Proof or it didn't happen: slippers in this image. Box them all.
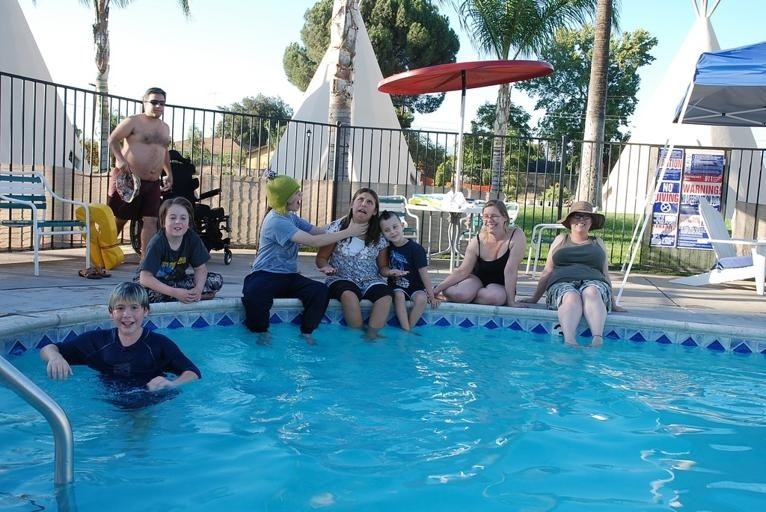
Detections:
[78,264,112,280]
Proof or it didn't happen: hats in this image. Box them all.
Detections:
[115,168,142,204]
[560,200,605,232]
[263,167,300,219]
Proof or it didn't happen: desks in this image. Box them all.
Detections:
[405,202,479,273]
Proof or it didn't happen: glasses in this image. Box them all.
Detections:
[146,98,166,107]
[570,212,592,220]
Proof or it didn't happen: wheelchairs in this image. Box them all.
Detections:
[129,150,232,265]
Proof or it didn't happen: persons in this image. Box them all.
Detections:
[378,209,442,336]
[104,86,175,263]
[242,169,370,345]
[315,186,413,343]
[433,199,531,309]
[39,280,202,412]
[129,198,225,304]
[515,200,632,346]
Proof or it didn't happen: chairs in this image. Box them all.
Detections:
[0,170,91,276]
[377,194,422,245]
[456,201,520,268]
[696,198,766,295]
[526,204,597,279]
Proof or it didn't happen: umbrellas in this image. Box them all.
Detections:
[375,59,555,272]
